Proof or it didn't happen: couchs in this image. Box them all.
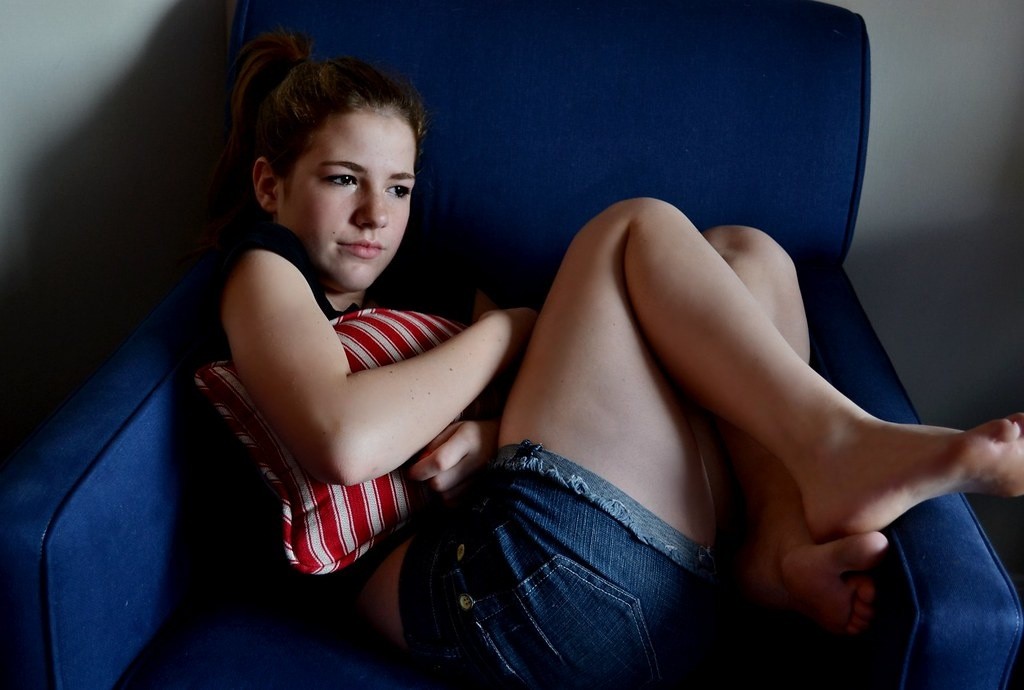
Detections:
[0,0,1024,690]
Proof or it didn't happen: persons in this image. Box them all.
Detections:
[181,32,1024,690]
